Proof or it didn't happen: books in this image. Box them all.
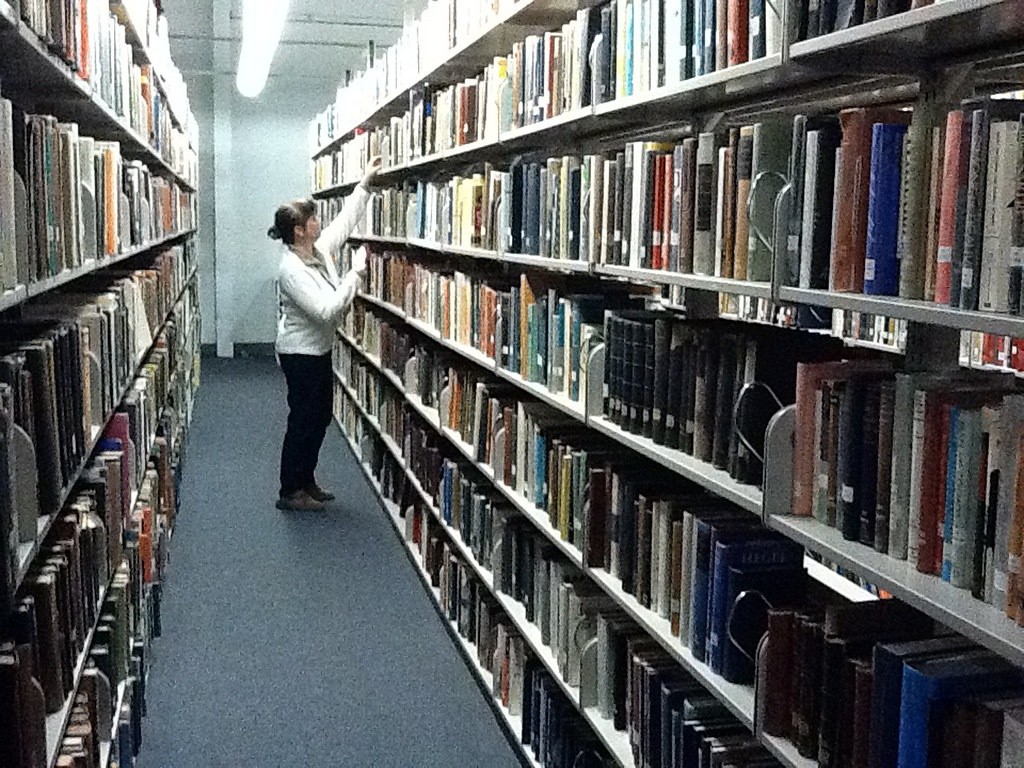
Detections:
[312,110,409,193]
[319,107,1024,318]
[311,0,527,157]
[408,0,944,158]
[0,0,203,768]
[333,241,1024,768]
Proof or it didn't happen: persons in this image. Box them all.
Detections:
[266,156,382,512]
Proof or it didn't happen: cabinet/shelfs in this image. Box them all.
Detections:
[0,0,200,768]
[309,0,1024,768]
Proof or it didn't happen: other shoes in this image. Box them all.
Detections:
[307,483,334,501]
[276,488,324,511]
